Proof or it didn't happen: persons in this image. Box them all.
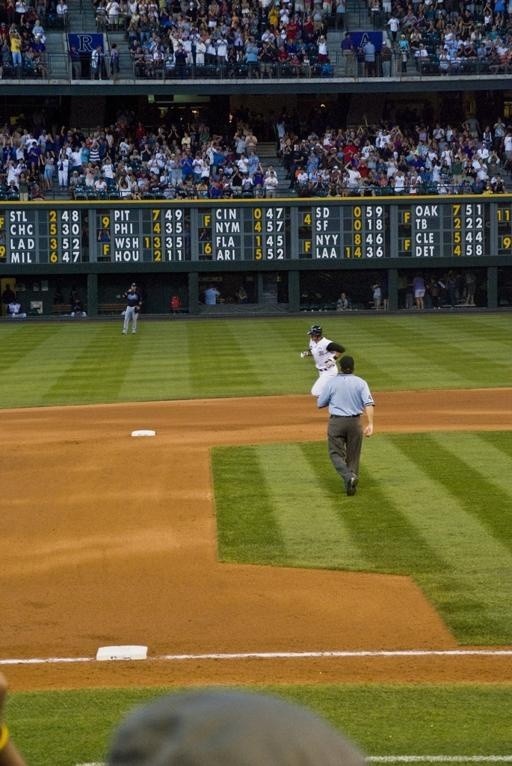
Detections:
[0,671,26,766]
[300,325,345,398]
[1,1,512,80]
[2,266,511,335]
[1,95,512,202]
[108,690,366,766]
[318,356,376,496]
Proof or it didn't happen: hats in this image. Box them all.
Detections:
[131,282,136,286]
[339,356,354,368]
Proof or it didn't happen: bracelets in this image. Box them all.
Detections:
[0,720,10,753]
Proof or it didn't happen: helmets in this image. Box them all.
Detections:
[306,325,322,336]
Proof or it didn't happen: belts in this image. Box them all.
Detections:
[319,368,327,371]
[330,414,359,419]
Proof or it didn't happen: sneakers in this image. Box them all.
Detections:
[121,330,137,334]
[347,472,359,496]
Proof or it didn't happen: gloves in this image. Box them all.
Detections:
[325,358,336,367]
[301,351,309,359]
